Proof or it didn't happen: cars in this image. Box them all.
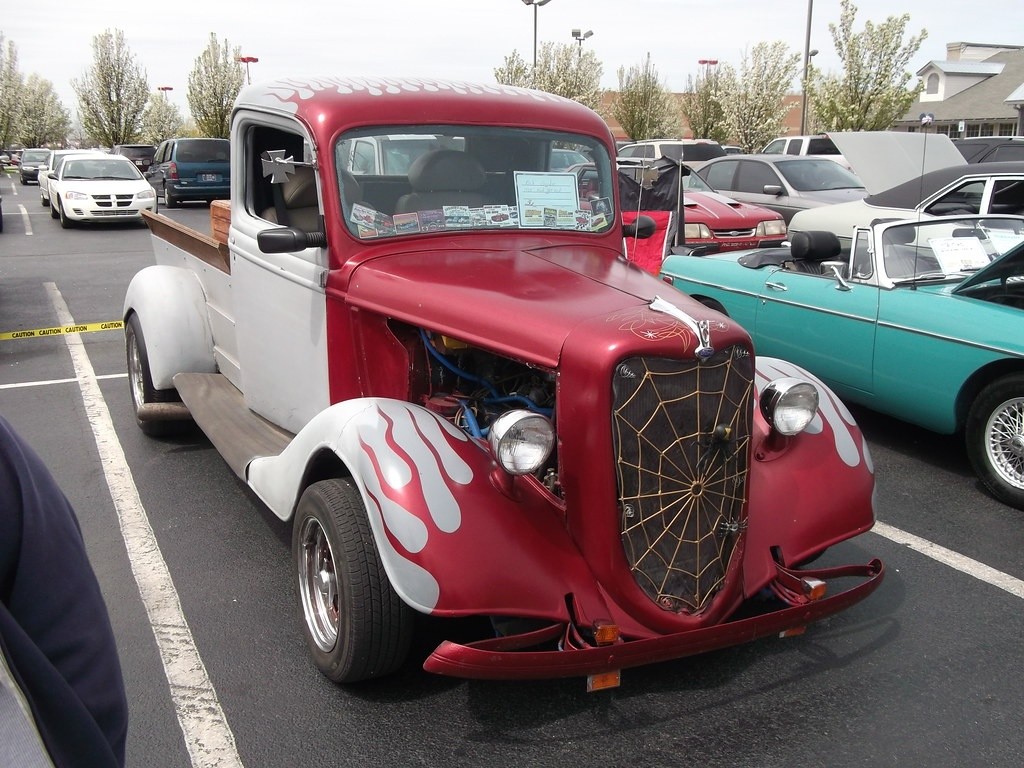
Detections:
[550,128,1024,234]
[45,152,159,228]
[785,161,1024,260]
[561,156,790,262]
[9,141,157,207]
[338,133,466,176]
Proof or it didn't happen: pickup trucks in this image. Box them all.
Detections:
[108,76,886,692]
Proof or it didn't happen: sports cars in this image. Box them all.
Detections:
[654,211,1024,511]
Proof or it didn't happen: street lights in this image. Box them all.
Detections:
[237,56,259,86]
[521,0,553,84]
[570,28,595,94]
[804,48,820,135]
[157,86,174,104]
[698,58,719,82]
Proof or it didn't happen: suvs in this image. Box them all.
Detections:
[142,137,230,210]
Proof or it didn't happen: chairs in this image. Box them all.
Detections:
[263,166,376,233]
[70,163,84,175]
[873,217,932,278]
[786,231,843,276]
[395,149,496,215]
